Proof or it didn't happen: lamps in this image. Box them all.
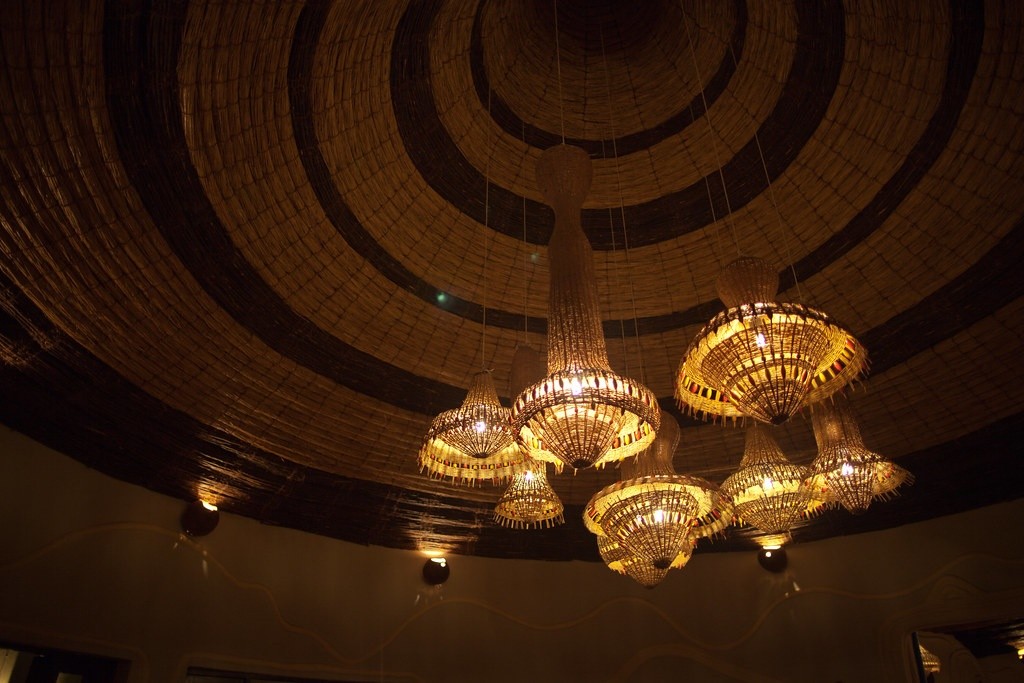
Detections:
[582,17,740,589]
[758,546,787,574]
[686,94,830,534]
[492,108,565,530]
[721,21,915,515]
[183,500,220,537]
[422,559,449,586]
[417,54,526,490]
[674,0,871,430]
[508,0,663,474]
